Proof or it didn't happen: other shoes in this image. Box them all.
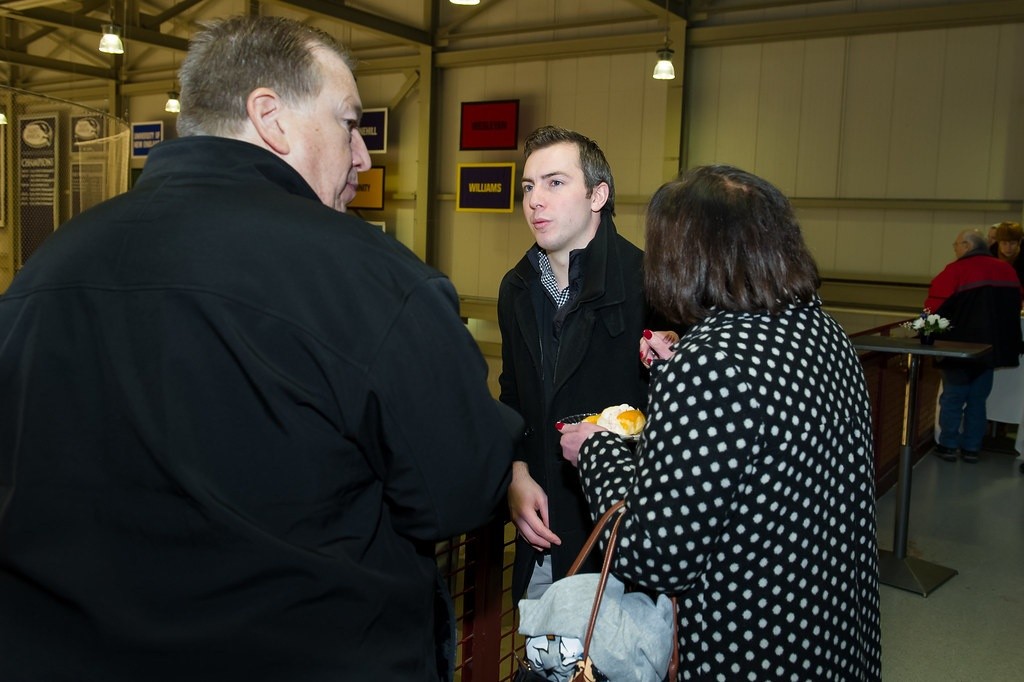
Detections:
[959,452,979,464]
[932,444,957,462]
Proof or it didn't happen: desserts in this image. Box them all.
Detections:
[582,404,647,437]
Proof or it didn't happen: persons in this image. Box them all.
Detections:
[554,166,881,681]
[0,17,518,682]
[924,221,1024,463]
[500,129,683,612]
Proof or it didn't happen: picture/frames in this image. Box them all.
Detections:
[455,162,515,213]
[345,165,385,210]
[458,99,518,152]
[356,107,388,154]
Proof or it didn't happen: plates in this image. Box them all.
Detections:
[558,412,601,426]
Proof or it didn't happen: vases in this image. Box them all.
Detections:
[920,331,937,345]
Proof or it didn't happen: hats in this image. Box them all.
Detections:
[987,222,1024,241]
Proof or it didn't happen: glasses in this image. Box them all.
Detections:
[952,241,967,248]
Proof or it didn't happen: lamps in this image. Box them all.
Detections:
[165,0,181,112]
[652,1,675,79]
[0,113,8,125]
[99,0,124,54]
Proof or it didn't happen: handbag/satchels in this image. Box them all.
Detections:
[511,500,679,682]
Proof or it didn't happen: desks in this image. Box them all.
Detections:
[844,343,992,597]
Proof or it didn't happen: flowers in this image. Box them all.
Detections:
[900,309,956,336]
